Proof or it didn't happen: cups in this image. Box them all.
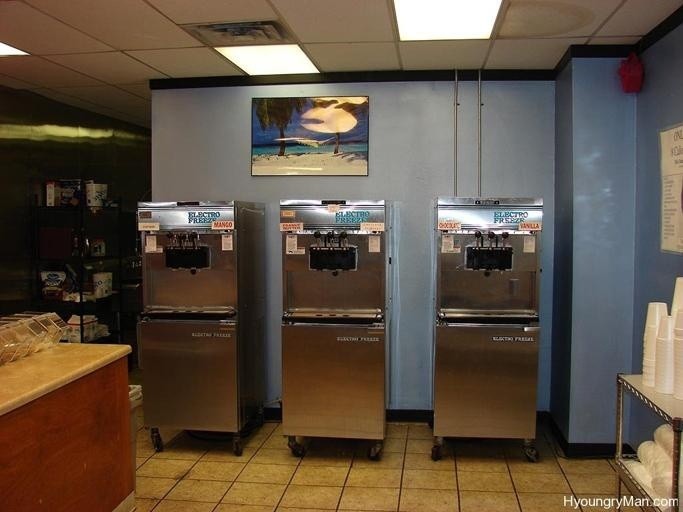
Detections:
[643,277,683,400]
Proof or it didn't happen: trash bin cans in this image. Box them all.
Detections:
[128,384,143,494]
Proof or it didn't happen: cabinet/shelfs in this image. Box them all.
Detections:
[28,191,145,366]
[614,372,683,512]
